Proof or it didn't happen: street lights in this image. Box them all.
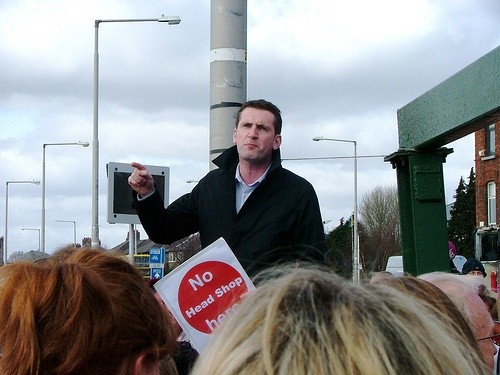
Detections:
[5,181,40,264]
[21,229,40,252]
[41,141,89,253]
[312,136,361,283]
[90,15,180,249]
[55,220,76,247]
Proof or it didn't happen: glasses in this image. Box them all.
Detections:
[477,321,500,346]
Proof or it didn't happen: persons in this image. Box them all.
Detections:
[0,248,197,375]
[190,258,500,375]
[128,99,325,286]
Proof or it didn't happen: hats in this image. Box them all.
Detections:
[462,258,487,277]
